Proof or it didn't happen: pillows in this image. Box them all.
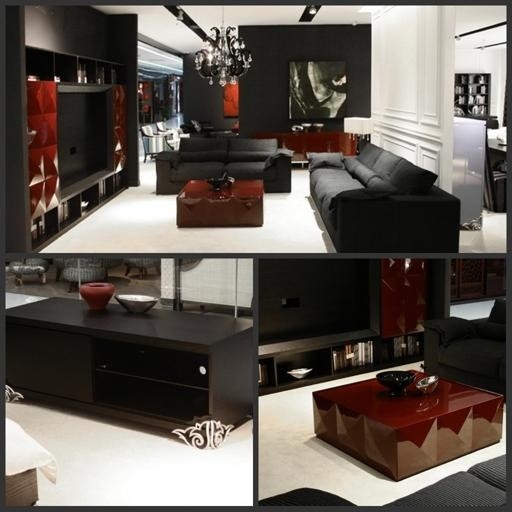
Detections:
[305,151,347,172]
[421,318,472,349]
[329,187,394,211]
[344,141,438,195]
[156,137,295,170]
[481,297,505,342]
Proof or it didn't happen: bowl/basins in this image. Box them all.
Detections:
[115,294,158,315]
[416,375,439,394]
[377,371,415,397]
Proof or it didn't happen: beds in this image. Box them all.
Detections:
[176,176,263,228]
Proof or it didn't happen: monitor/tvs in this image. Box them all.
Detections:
[57,92,108,189]
[258,258,381,357]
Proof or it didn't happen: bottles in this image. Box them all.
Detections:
[78,63,88,84]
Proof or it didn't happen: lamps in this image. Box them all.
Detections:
[344,117,376,156]
[193,5,253,87]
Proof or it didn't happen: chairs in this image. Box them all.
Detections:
[482,125,506,212]
[155,121,179,140]
[140,125,175,163]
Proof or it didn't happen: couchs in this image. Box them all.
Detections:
[257,453,507,507]
[155,148,292,195]
[309,155,461,253]
[423,318,506,402]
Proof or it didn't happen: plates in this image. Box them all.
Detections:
[287,368,313,379]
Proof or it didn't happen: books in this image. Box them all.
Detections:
[258,363,266,384]
[77,63,87,83]
[332,341,373,371]
[30,216,46,241]
[58,202,70,223]
[394,336,420,358]
[27,74,38,82]
[455,74,486,115]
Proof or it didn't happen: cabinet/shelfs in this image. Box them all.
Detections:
[29,164,130,253]
[5,295,253,451]
[454,73,491,120]
[26,45,126,93]
[258,322,427,397]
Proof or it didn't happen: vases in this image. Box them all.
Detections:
[79,282,115,314]
